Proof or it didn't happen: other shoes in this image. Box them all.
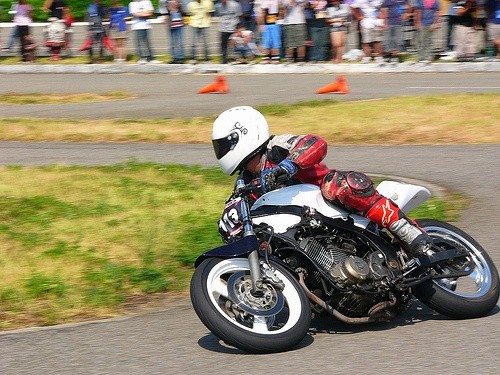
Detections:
[410,235,431,258]
[137,58,148,65]
[169,58,186,65]
[114,58,127,63]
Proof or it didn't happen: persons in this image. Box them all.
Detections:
[212,104,433,257]
[0,0,500,73]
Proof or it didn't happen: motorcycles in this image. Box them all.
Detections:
[189,171,500,356]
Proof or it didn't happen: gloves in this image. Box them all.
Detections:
[260,165,291,194]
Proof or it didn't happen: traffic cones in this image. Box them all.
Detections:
[198,74,231,97]
[315,74,352,94]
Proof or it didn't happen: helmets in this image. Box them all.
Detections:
[209,104,270,177]
